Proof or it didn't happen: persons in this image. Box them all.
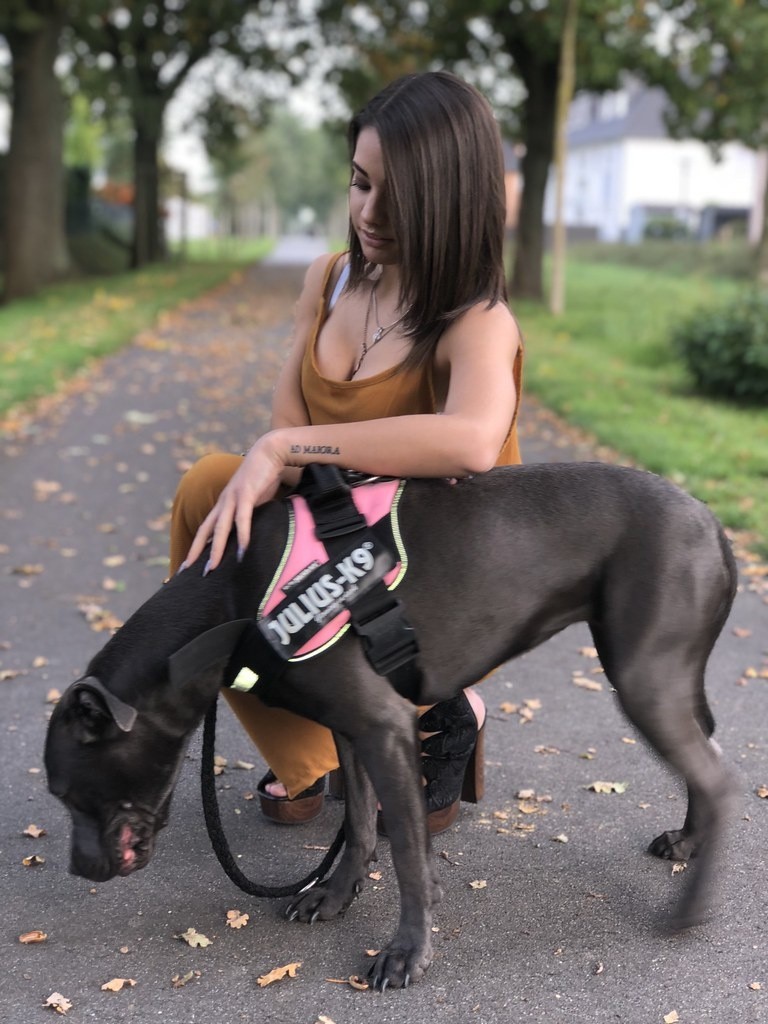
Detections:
[164,71,524,838]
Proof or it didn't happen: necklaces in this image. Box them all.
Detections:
[349,264,420,378]
[372,271,418,341]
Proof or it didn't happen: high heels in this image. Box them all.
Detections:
[374,689,489,840]
[257,760,347,825]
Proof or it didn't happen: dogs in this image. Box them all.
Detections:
[41,462,741,997]
